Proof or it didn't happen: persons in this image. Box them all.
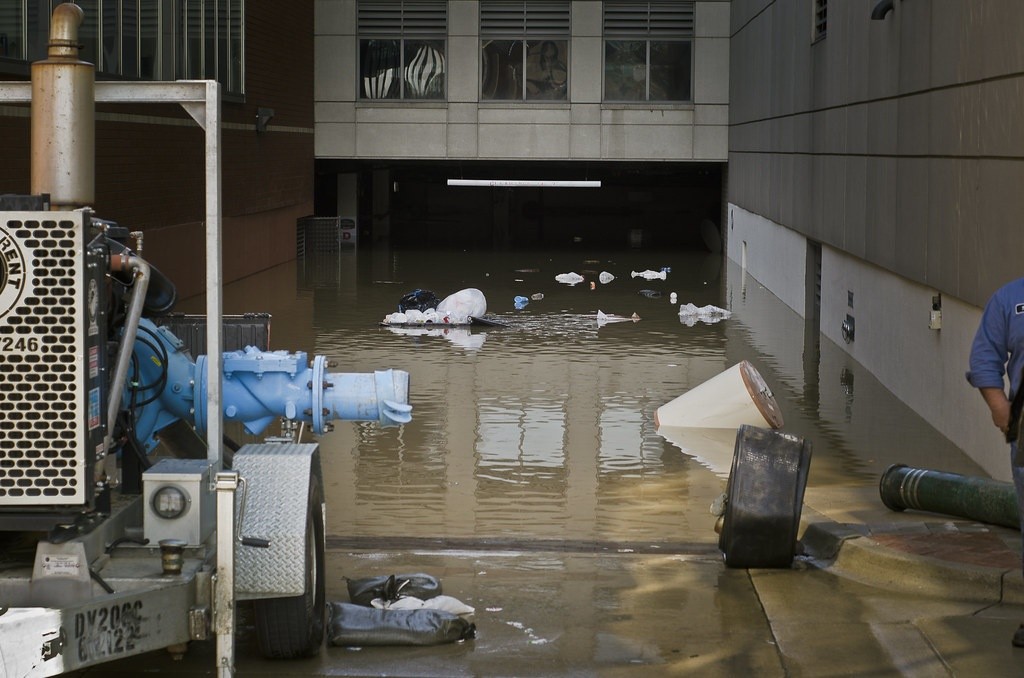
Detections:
[964,275,1024,648]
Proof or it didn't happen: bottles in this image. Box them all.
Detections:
[531,293,544,300]
[514,303,529,309]
[514,296,529,303]
[660,267,671,272]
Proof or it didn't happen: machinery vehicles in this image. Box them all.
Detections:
[0,1,419,678]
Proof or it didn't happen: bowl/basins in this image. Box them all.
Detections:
[715,424,812,569]
